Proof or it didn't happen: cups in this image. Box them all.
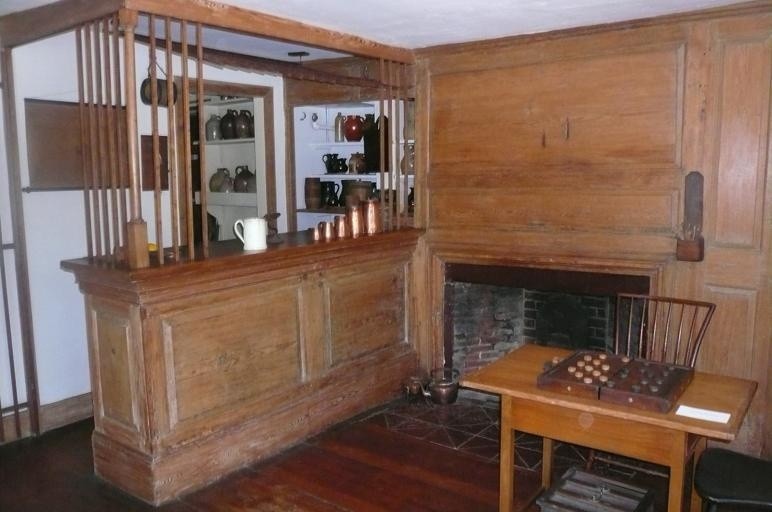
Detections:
[232,217,267,251]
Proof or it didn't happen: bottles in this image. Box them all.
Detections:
[309,198,382,242]
[399,142,414,175]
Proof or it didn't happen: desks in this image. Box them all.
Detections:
[458,342,758,512]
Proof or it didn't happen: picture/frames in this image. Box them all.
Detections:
[23,98,133,192]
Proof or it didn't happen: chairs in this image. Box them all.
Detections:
[585,291,716,489]
[694,448,772,512]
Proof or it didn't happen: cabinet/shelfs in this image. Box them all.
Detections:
[195,97,268,241]
[283,54,417,232]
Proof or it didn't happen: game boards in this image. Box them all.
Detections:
[536,349,696,413]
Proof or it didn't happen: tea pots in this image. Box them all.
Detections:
[413,367,462,406]
[403,369,431,401]
[205,108,255,140]
[209,164,257,192]
[305,177,415,209]
[322,111,389,176]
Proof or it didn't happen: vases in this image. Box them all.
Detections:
[206,108,257,193]
[304,111,416,213]
